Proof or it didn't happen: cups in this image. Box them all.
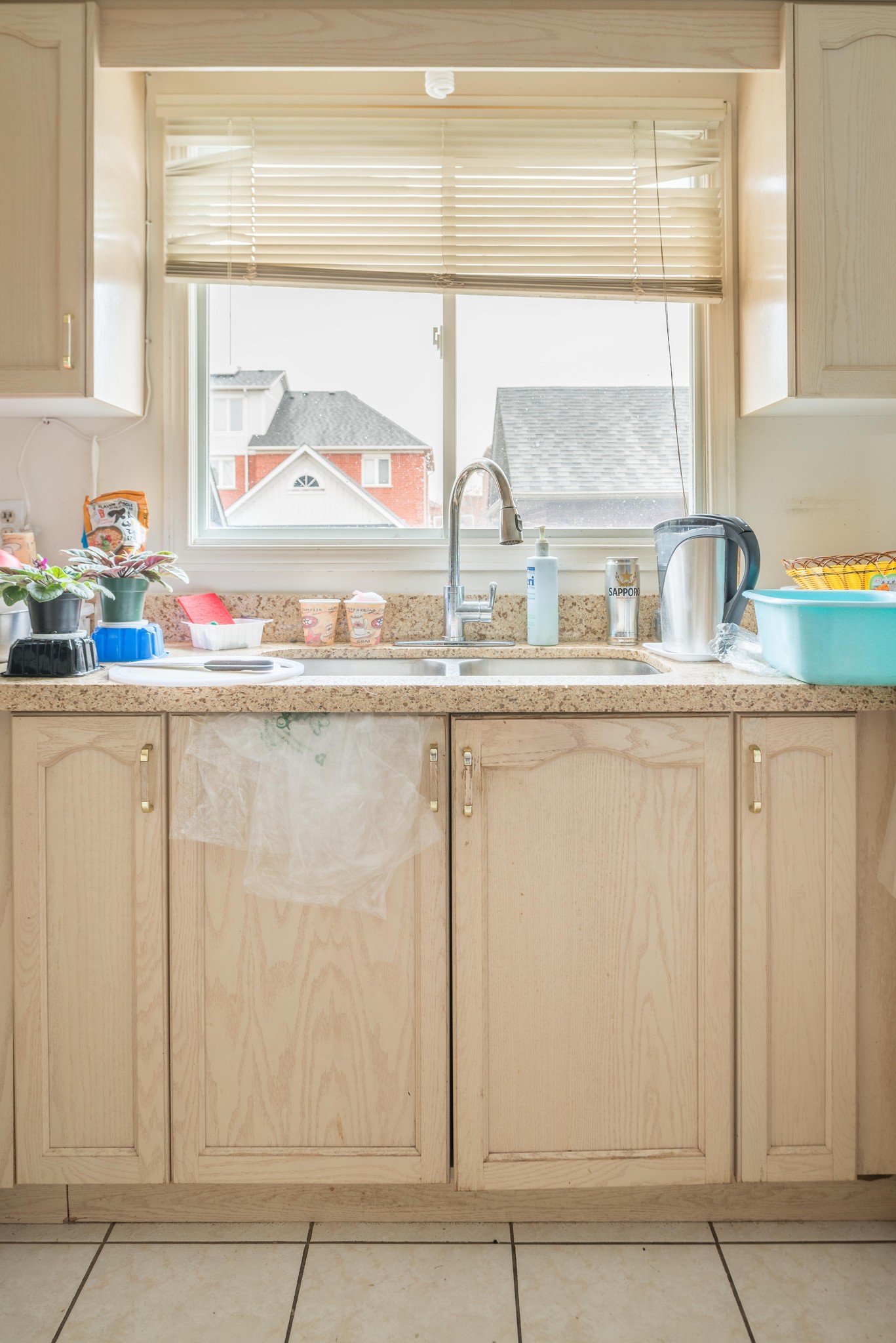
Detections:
[2,532,37,567]
[605,557,640,646]
[343,599,387,647]
[299,599,341,646]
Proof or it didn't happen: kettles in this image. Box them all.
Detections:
[653,514,760,654]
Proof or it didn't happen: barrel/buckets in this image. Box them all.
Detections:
[0,576,31,663]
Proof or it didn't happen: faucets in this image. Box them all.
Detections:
[393,458,523,647]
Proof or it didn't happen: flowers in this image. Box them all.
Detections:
[0,553,116,607]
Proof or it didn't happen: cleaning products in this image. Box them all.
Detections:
[527,525,558,646]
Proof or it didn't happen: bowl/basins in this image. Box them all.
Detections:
[0,636,106,678]
[742,589,896,685]
[91,623,170,662]
[181,617,273,651]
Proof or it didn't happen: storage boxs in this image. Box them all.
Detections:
[188,617,274,651]
[742,590,896,687]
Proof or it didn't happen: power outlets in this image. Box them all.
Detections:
[0,499,25,548]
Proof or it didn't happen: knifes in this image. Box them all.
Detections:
[114,660,274,671]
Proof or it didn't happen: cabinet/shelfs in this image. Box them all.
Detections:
[12,712,856,1187]
[0,0,146,416]
[739,0,896,418]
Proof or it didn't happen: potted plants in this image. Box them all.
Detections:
[26,591,82,634]
[59,546,189,622]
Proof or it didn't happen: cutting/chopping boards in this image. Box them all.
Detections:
[108,656,305,687]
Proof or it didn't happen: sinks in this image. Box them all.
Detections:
[452,658,663,676]
[274,658,454,676]
